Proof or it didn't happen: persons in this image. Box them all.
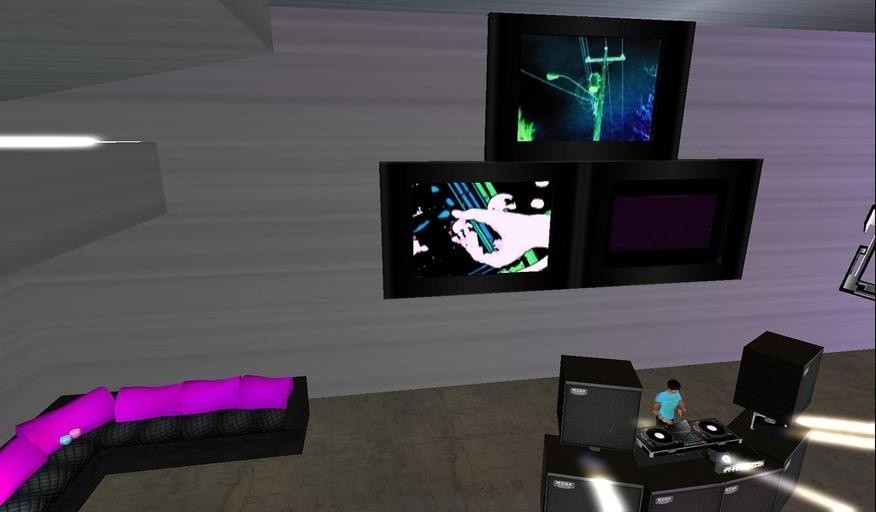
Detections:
[649,378,688,428]
[449,206,550,270]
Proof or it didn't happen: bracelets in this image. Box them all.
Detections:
[657,414,664,421]
